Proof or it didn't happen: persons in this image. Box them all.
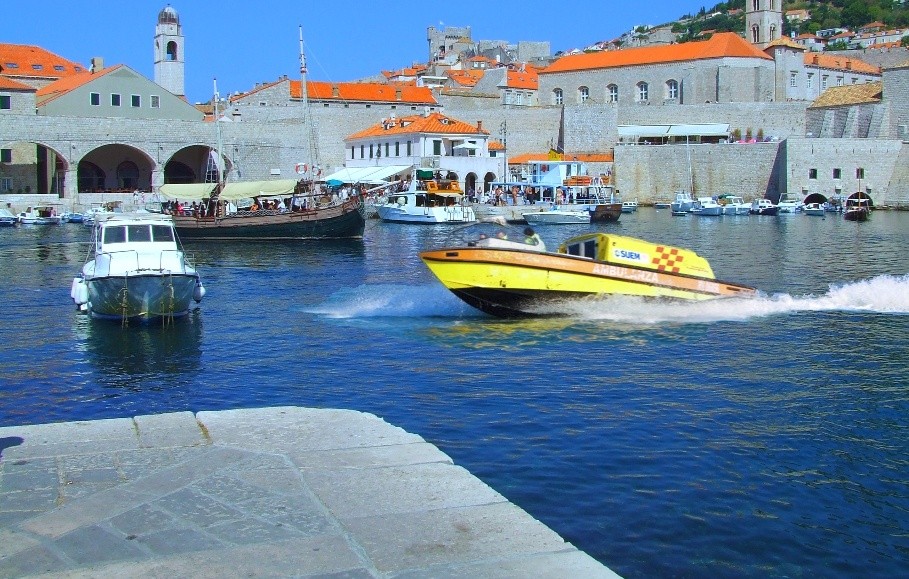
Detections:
[564,186,571,204]
[522,226,540,246]
[511,186,519,206]
[476,186,483,204]
[395,180,424,191]
[523,185,533,205]
[249,199,316,215]
[467,185,475,203]
[340,185,377,200]
[535,189,551,201]
[132,188,144,205]
[494,228,509,242]
[433,202,438,207]
[162,199,203,216]
[556,186,562,205]
[490,185,507,206]
[448,198,453,206]
[44,207,55,217]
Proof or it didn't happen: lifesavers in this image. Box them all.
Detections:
[295,162,309,175]
[311,165,321,176]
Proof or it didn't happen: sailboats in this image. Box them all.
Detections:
[845,165,870,222]
[143,24,404,240]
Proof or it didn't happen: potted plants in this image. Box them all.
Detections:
[755,128,763,142]
[746,127,752,142]
[731,128,742,142]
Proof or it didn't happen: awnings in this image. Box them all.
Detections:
[319,165,413,184]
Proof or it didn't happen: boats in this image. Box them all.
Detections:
[671,193,694,212]
[718,195,752,216]
[62,207,105,226]
[418,221,758,318]
[690,196,722,216]
[621,202,670,212]
[16,206,60,225]
[778,192,804,214]
[522,210,591,224]
[487,149,623,222]
[672,212,686,217]
[803,202,825,216]
[371,190,476,224]
[751,197,781,215]
[0,200,18,226]
[70,211,206,325]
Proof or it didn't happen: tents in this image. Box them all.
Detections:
[160,178,297,216]
